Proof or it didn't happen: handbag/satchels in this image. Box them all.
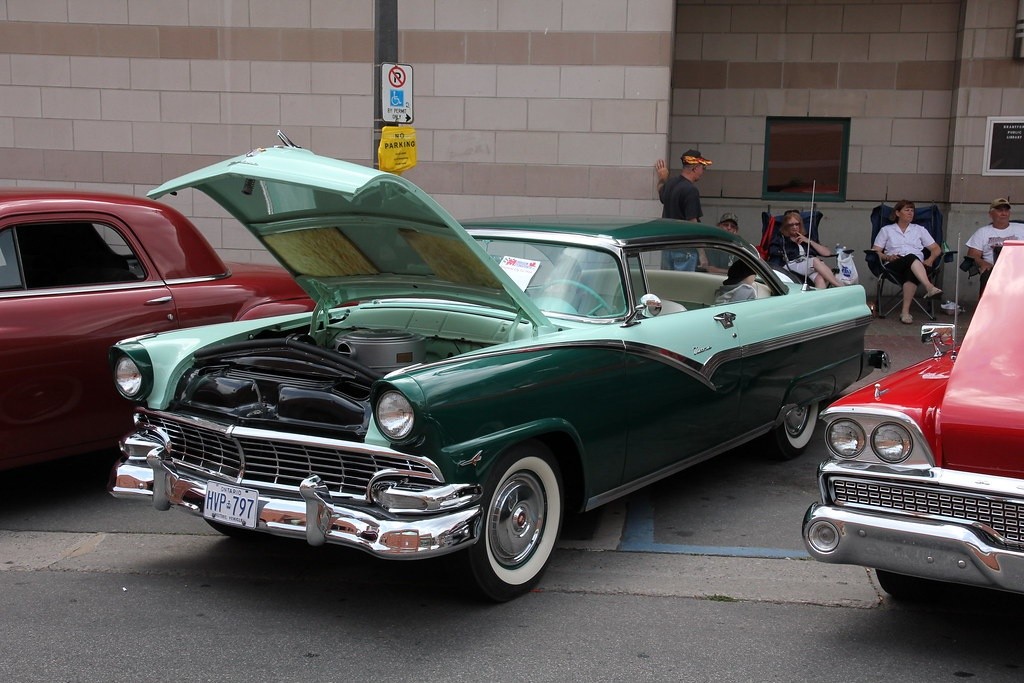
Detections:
[836,251,859,285]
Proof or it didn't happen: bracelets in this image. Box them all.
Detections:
[810,239,812,245]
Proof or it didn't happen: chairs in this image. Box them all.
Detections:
[761,204,855,290]
[863,201,958,322]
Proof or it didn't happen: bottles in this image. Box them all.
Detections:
[835,243,846,255]
[942,241,951,253]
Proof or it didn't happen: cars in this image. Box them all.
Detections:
[800,238,1024,608]
[0,188,361,471]
[106,129,891,605]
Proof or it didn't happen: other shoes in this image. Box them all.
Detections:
[899,313,914,324]
[923,287,944,301]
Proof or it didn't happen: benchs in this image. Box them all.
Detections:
[577,268,770,312]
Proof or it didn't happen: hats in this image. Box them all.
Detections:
[721,212,739,228]
[681,149,713,166]
[989,198,1011,209]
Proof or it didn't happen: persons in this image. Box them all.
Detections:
[966,197,1024,303]
[769,213,845,290]
[655,150,713,272]
[872,199,944,324]
[697,213,739,274]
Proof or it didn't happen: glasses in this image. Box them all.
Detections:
[783,210,799,217]
